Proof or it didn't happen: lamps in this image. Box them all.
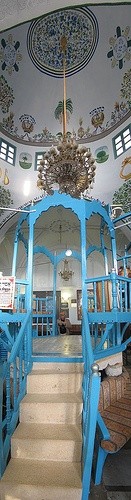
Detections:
[58,260,74,281]
[34,139,96,201]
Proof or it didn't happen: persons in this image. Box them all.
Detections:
[49,311,73,335]
[118,265,131,310]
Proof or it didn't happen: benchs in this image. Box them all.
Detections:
[98,363,131,454]
